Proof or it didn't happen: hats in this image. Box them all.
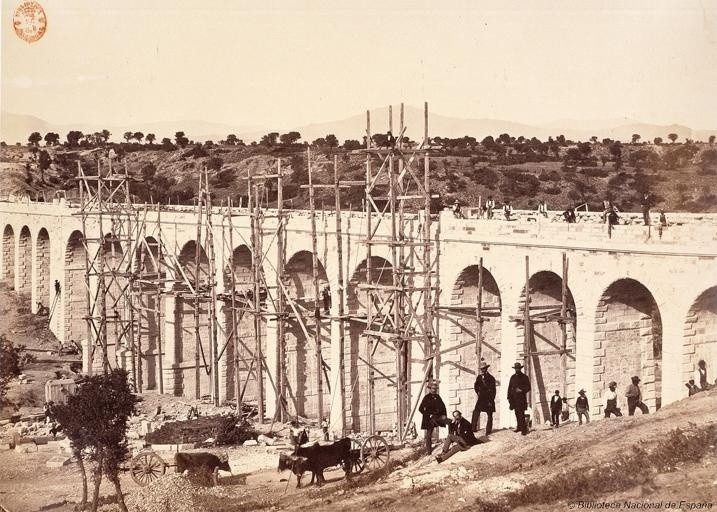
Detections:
[553,389,560,394]
[427,383,439,389]
[688,379,695,385]
[578,388,587,395]
[608,381,617,389]
[697,360,706,366]
[630,375,642,383]
[478,362,490,370]
[511,362,524,371]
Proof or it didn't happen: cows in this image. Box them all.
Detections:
[169,450,234,478]
[276,452,343,487]
[291,437,352,488]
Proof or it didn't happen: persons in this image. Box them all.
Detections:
[624,376,649,416]
[55,279,59,296]
[436,409,484,463]
[507,363,530,435]
[562,188,667,227]
[685,380,702,396]
[471,361,496,436]
[502,200,512,221]
[452,200,465,220]
[693,360,713,391]
[536,199,548,218]
[603,382,622,418]
[321,286,329,313]
[576,389,590,426]
[485,196,494,219]
[419,382,452,456]
[549,390,562,428]
[561,397,575,421]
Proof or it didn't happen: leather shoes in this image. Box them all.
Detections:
[549,423,560,430]
[514,428,529,438]
[472,428,492,437]
[425,451,443,465]
